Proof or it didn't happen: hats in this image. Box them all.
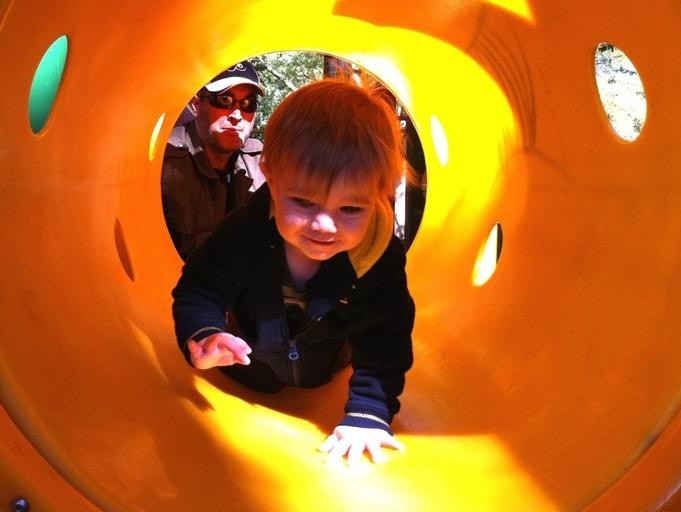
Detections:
[205,61,265,96]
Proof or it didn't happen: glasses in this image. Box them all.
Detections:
[201,94,257,112]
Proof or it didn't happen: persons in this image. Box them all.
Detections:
[172,64,416,467]
[161,60,427,264]
